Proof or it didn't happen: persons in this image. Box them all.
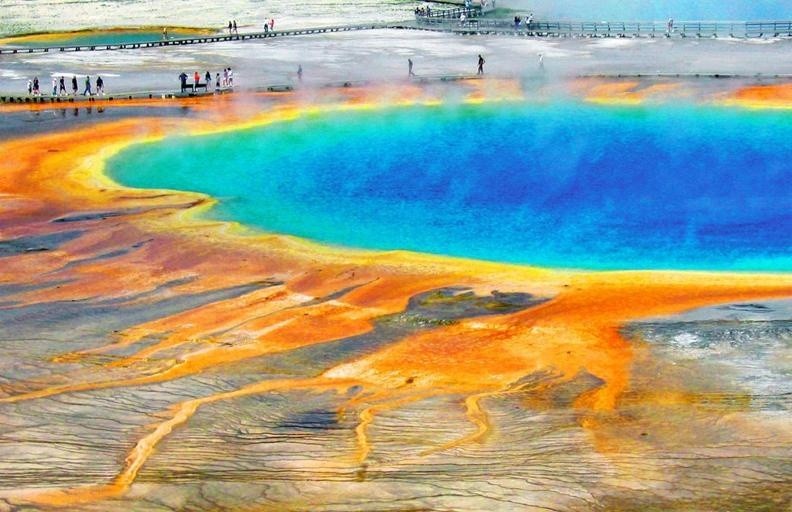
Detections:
[460,13,465,28]
[228,21,232,35]
[270,19,274,31]
[205,67,233,89]
[27,80,32,95]
[233,20,237,34]
[264,24,268,32]
[84,75,91,96]
[59,76,68,96]
[408,59,413,77]
[477,54,484,75]
[537,53,544,73]
[415,6,430,17]
[163,28,168,40]
[95,75,105,96]
[179,71,187,92]
[33,75,40,96]
[512,13,533,31]
[194,71,200,91]
[297,65,302,75]
[667,17,673,32]
[51,78,57,96]
[72,75,79,95]
[464,0,495,11]
